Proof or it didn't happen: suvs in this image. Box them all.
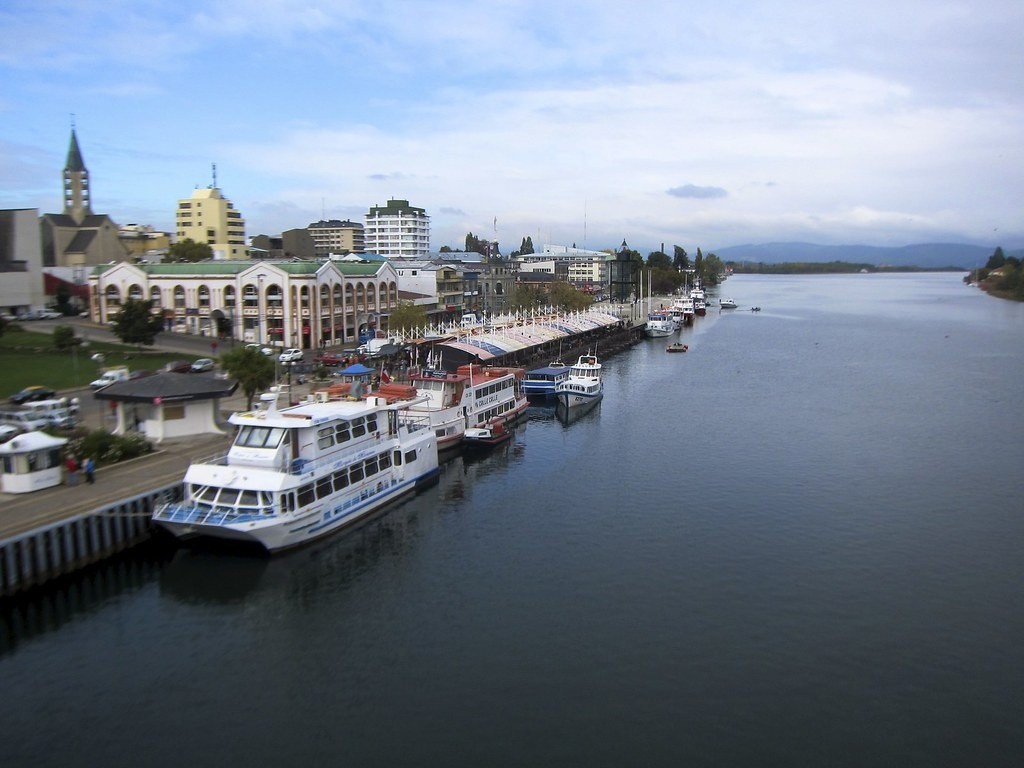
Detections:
[278,349,304,363]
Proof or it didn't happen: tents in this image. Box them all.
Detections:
[338,364,376,386]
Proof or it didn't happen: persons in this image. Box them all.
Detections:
[320,339,326,350]
[344,355,350,368]
[212,341,218,355]
[64,453,95,486]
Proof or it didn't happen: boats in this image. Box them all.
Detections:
[752,307,761,312]
[462,415,513,446]
[645,281,711,339]
[522,362,572,406]
[389,364,530,452]
[557,355,603,407]
[720,297,737,308]
[666,342,687,353]
[557,393,604,427]
[151,381,443,555]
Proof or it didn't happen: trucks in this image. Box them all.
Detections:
[358,339,389,353]
[359,329,386,344]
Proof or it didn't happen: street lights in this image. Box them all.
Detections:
[282,363,294,406]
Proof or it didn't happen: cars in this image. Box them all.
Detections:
[0,419,18,443]
[38,409,76,430]
[11,386,57,405]
[191,359,216,374]
[593,293,610,302]
[247,344,273,356]
[0,307,63,322]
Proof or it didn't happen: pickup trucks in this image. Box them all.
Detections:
[313,351,345,367]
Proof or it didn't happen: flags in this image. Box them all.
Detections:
[426,351,430,362]
[382,366,390,385]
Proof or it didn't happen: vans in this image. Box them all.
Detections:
[1,410,48,434]
[460,314,477,327]
[89,366,130,390]
